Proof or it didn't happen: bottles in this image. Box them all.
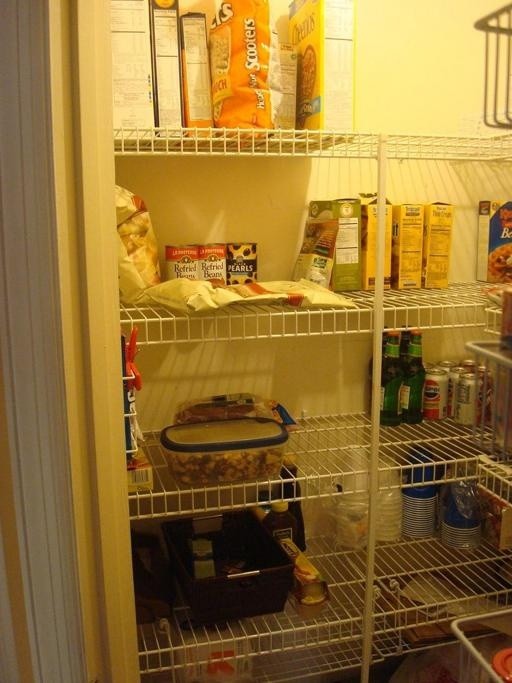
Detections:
[370,324,424,424]
[265,501,298,547]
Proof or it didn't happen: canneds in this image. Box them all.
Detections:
[198,244,227,289]
[419,359,495,427]
[227,243,258,286]
[165,243,198,283]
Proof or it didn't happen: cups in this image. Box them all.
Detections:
[338,442,487,555]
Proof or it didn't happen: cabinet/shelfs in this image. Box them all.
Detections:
[112,125,512,683]
